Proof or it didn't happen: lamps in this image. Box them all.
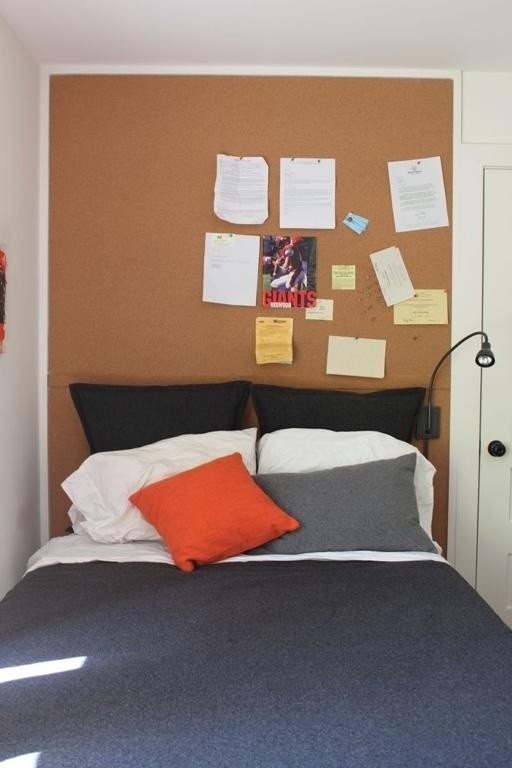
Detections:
[416,330,496,439]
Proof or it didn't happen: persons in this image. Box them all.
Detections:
[270,236,306,292]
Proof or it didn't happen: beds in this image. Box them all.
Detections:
[0,522,512,767]
